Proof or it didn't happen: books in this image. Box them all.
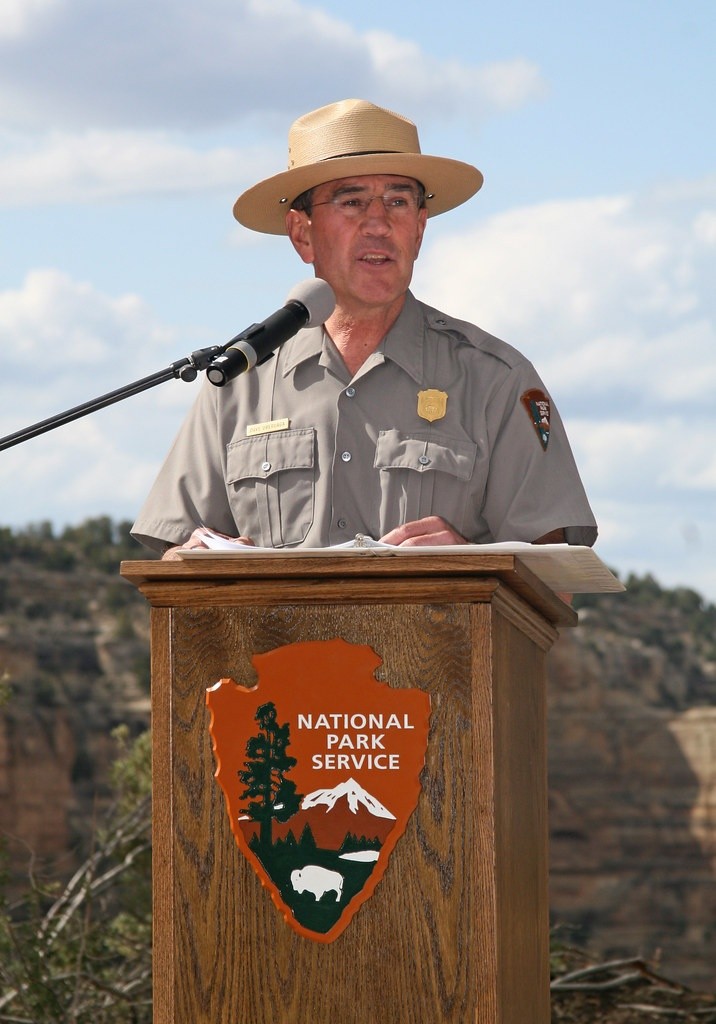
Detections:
[176,522,628,594]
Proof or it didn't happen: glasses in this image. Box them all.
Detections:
[297,188,419,221]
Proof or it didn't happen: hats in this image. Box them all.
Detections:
[232,99,484,236]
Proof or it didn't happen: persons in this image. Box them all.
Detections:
[130,96,600,557]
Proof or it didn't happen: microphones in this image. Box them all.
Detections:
[206,277,335,387]
